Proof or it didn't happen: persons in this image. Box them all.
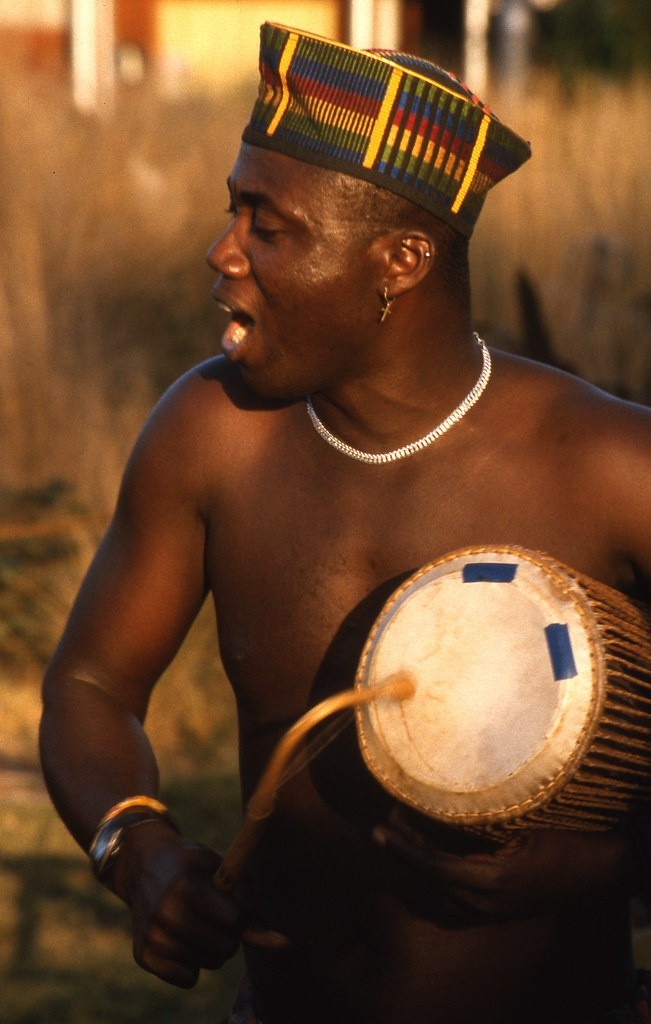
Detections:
[36,19,650,1023]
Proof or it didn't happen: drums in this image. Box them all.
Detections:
[351,543,651,850]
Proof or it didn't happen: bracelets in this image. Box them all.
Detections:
[98,795,169,824]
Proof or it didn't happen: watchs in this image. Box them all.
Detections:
[89,810,182,885]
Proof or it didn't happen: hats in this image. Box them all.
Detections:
[237,20,535,238]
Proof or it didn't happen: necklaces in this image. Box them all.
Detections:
[307,331,492,464]
